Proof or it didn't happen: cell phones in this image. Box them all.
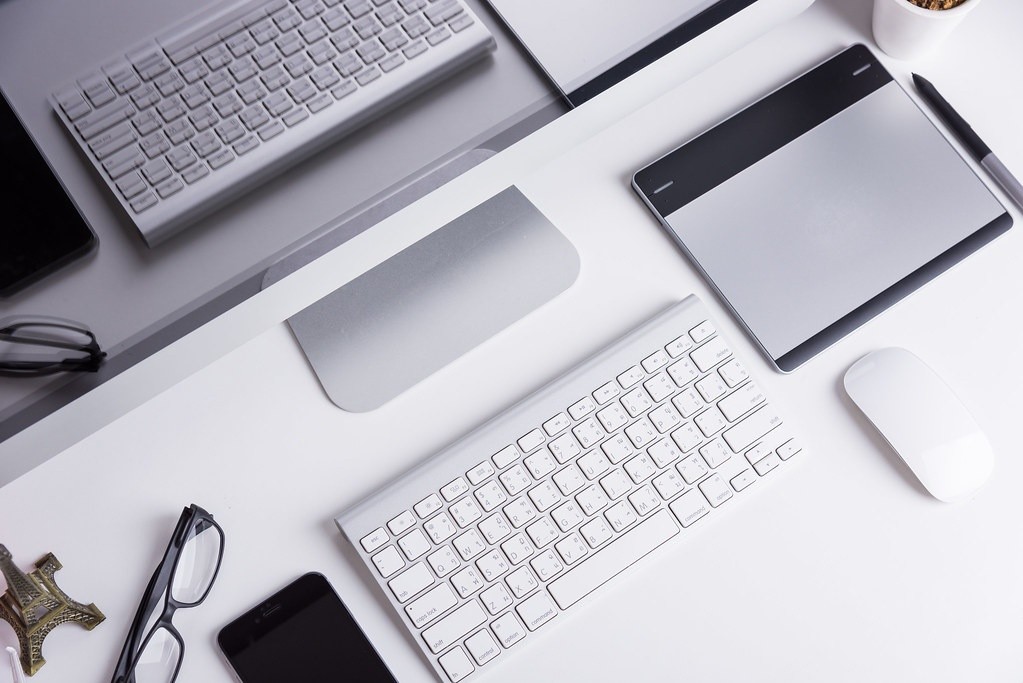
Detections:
[0,88,101,302]
[218,571,399,683]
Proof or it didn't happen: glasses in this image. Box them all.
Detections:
[0,314,107,378]
[110,504,226,683]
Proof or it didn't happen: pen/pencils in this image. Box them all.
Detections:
[910,70,1023,211]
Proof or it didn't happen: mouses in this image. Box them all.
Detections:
[844,347,996,505]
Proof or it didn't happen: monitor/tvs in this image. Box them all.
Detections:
[0,0,815,490]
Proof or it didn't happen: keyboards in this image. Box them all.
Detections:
[334,292,800,682]
[45,0,499,249]
